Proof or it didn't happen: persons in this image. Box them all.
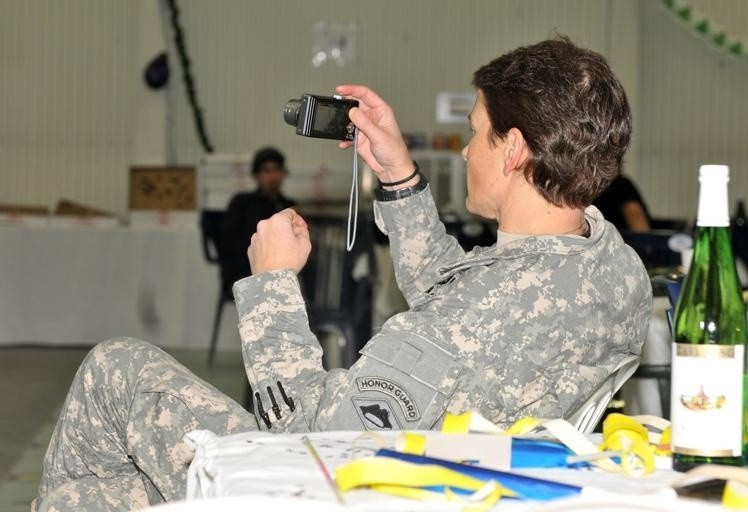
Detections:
[220,147,301,301]
[593,173,659,269]
[30,29,653,512]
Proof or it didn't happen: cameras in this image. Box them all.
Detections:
[284,93,359,141]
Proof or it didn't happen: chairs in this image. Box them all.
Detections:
[198,207,316,367]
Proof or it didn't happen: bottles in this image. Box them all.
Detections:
[671,165,748,472]
